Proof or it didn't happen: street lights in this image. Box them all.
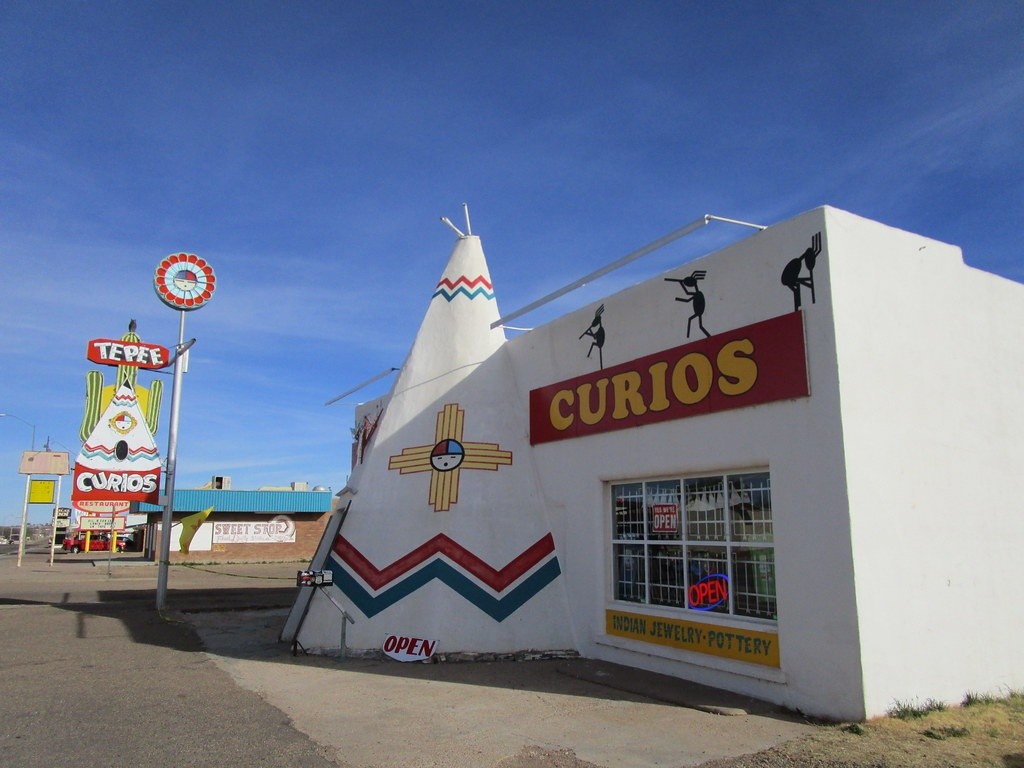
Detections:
[0,412,36,451]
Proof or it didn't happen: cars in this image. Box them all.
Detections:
[62,531,127,554]
